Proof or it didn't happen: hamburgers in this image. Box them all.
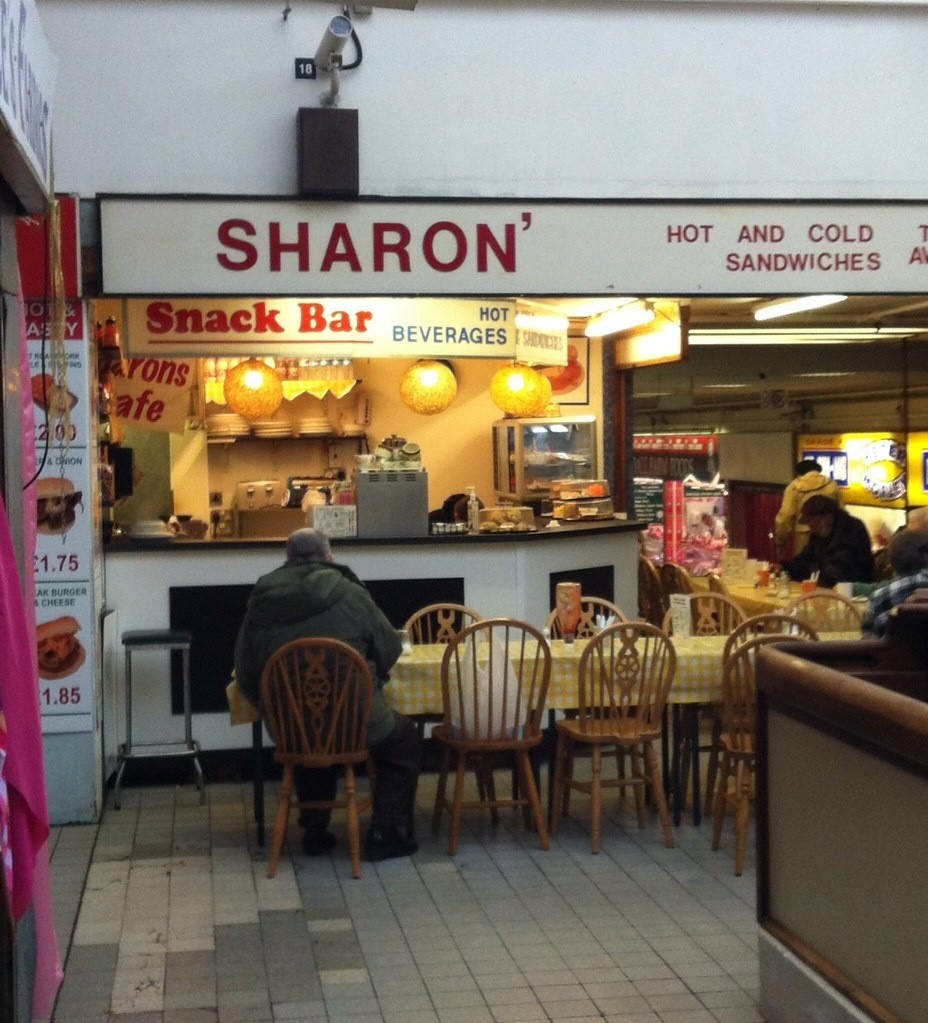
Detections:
[34,478,84,535]
[35,616,87,680]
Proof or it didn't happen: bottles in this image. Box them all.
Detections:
[765,573,779,596]
[399,631,412,655]
[779,571,790,598]
[376,433,422,470]
[556,582,581,650]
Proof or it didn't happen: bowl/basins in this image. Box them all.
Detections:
[132,520,164,533]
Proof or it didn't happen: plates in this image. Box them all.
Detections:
[122,530,177,538]
[206,413,333,439]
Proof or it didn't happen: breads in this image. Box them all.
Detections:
[481,510,528,532]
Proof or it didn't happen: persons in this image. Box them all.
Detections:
[859,506,928,641]
[774,460,844,559]
[702,515,724,539]
[428,494,483,524]
[768,495,877,590]
[233,527,417,866]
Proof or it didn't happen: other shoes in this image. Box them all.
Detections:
[363,832,418,862]
[302,830,336,854]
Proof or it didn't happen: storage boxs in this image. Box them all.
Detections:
[239,508,308,538]
[540,478,615,521]
[310,505,357,538]
[479,506,534,525]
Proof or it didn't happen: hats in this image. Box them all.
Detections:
[796,495,837,525]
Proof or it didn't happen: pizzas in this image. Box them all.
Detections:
[531,360,584,394]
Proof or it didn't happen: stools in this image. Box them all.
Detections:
[112,629,208,812]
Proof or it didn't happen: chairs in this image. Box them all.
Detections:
[259,638,378,879]
[663,592,752,826]
[551,621,676,854]
[660,562,698,620]
[782,591,859,631]
[639,554,665,623]
[709,573,738,620]
[404,603,490,646]
[432,618,551,856]
[703,612,817,878]
[545,596,633,638]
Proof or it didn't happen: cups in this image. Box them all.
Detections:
[593,627,611,649]
[353,454,376,469]
[836,582,853,601]
[801,580,817,599]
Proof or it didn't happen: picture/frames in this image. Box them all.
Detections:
[528,335,590,405]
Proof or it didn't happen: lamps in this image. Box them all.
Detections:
[752,294,848,321]
[223,357,285,420]
[583,299,655,337]
[399,358,457,415]
[489,366,552,418]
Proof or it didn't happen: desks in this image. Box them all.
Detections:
[691,576,869,632]
[225,633,864,845]
